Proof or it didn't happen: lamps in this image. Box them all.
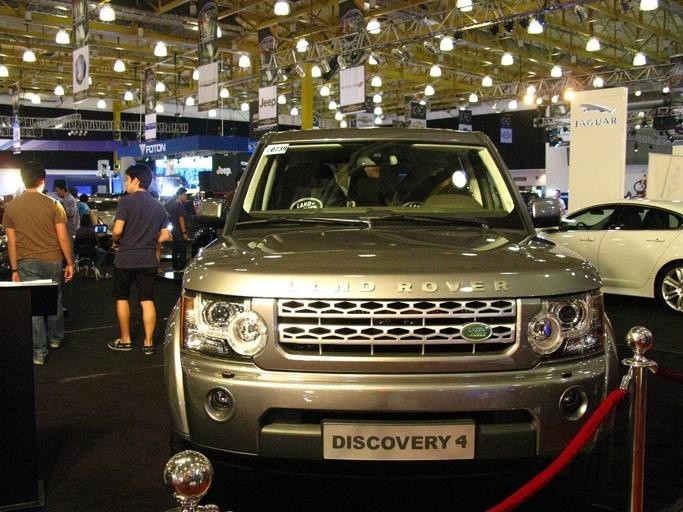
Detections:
[0,0,683,130]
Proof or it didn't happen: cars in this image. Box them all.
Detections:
[0,223,12,277]
[518,188,567,229]
[75,192,122,236]
[534,200,682,313]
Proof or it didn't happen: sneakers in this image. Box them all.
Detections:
[140,343,155,355]
[49,338,62,348]
[107,339,134,351]
[33,355,48,365]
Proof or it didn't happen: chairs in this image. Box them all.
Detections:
[284,165,348,207]
[72,236,95,282]
[390,162,472,208]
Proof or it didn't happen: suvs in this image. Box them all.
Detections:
[163,127,622,484]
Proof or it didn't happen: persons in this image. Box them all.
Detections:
[106,165,171,356]
[53,178,242,280]
[320,162,386,207]
[555,190,566,215]
[2,162,74,365]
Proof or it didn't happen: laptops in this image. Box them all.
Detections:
[94,225,107,235]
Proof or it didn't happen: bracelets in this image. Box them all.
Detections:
[10,268,17,273]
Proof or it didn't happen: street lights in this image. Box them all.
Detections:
[97,159,120,194]
